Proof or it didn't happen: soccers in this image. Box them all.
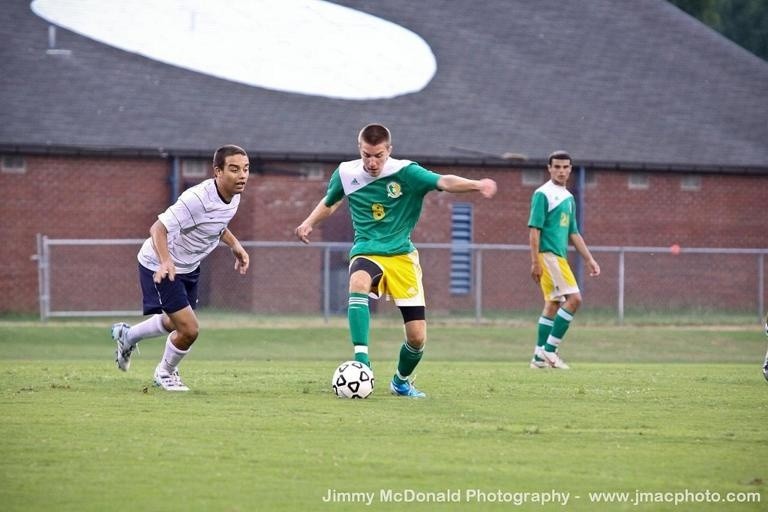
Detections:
[332,360,374,399]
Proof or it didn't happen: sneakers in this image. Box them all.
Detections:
[529,344,572,371]
[153,364,193,392]
[111,322,135,372]
[390,374,427,398]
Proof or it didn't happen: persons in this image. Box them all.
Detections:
[294,122,498,397]
[524,149,601,371]
[110,143,250,391]
